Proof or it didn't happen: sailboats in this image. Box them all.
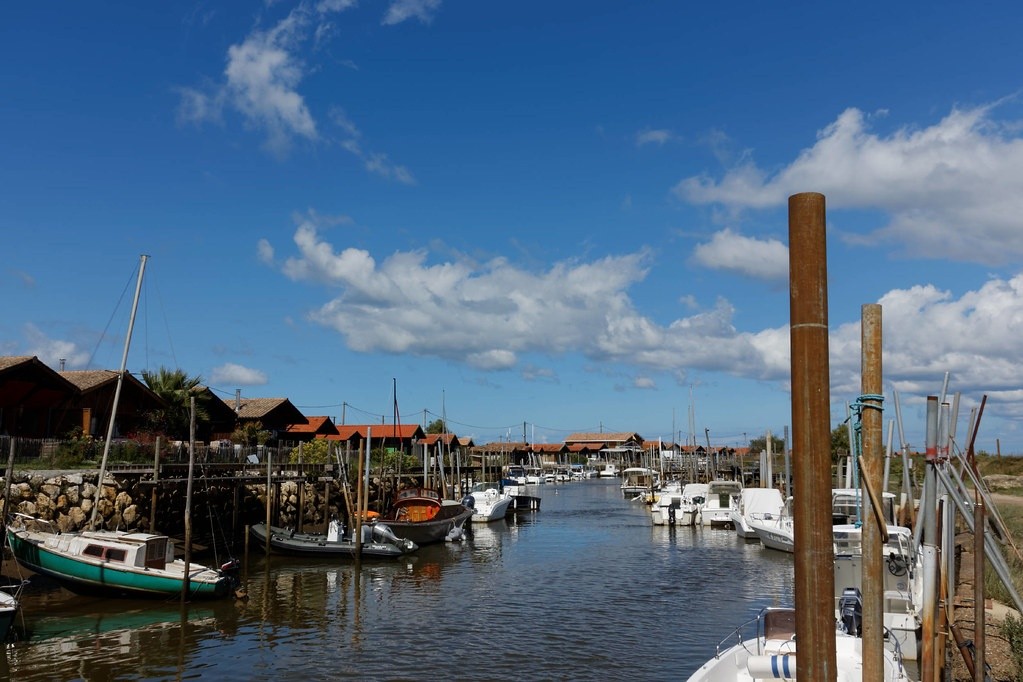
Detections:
[4,255,233,598]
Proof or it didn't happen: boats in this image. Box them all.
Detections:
[253,522,419,559]
[352,497,474,543]
[746,491,913,559]
[621,467,660,497]
[639,435,788,539]
[685,602,911,682]
[498,423,619,509]
[835,589,922,662]
[457,490,513,523]
